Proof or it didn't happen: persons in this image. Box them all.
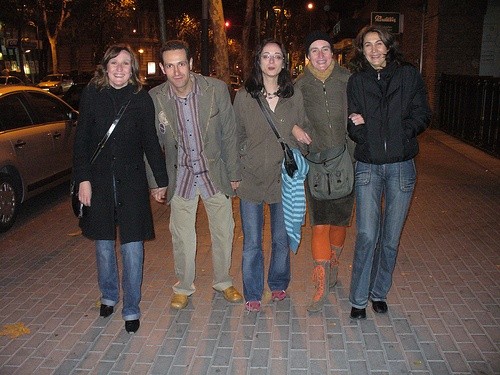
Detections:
[71,43,168,334]
[143,41,243,308]
[347,25,432,321]
[233,39,313,314]
[291,31,365,313]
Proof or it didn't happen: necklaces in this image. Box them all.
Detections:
[266,91,276,98]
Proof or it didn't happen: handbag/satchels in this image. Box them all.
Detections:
[282,143,298,177]
[71,179,87,218]
[305,143,355,200]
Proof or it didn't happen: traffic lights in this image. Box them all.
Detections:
[225,20,230,30]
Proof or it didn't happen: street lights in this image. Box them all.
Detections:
[28,21,41,62]
[272,4,293,46]
[308,4,313,32]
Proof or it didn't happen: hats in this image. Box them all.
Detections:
[305,31,334,58]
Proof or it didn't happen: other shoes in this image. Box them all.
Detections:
[351,307,366,320]
[368,296,387,313]
[125,318,140,334]
[212,286,243,303]
[170,293,192,307]
[272,289,286,302]
[99,304,114,318]
[246,300,261,312]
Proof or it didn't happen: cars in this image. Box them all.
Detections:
[0,85,80,233]
[0,76,25,87]
[230,75,243,90]
[37,74,75,94]
[142,78,164,90]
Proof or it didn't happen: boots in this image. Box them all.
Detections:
[306,259,331,311]
[329,241,344,287]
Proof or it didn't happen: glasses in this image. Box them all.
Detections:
[260,52,285,61]
